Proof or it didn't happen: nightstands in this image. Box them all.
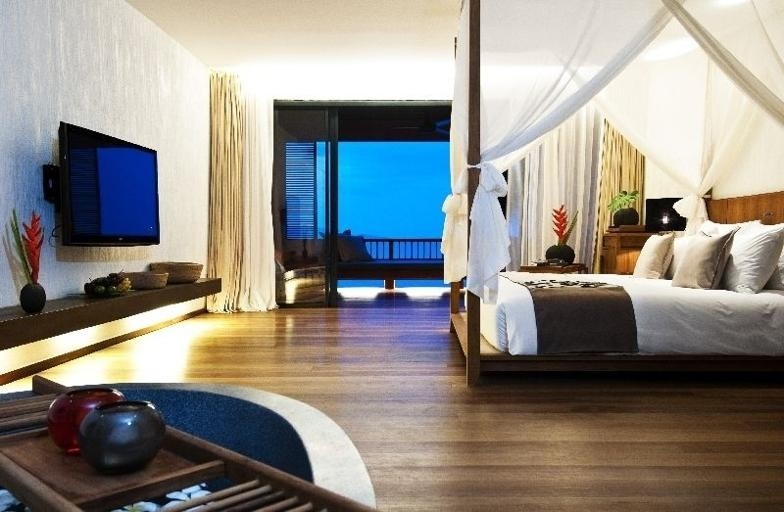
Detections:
[601,231,659,273]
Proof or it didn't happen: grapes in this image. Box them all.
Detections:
[107,273,132,296]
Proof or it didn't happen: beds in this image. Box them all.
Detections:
[448,190,783,389]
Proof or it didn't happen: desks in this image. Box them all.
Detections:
[517,264,589,274]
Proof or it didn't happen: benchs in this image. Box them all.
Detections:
[330,238,444,290]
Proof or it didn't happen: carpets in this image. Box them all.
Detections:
[0,381,377,512]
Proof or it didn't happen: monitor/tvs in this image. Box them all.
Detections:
[57,120,161,247]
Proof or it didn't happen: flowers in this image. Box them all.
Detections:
[7,207,45,285]
[551,204,580,246]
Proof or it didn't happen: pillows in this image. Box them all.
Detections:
[337,235,370,262]
[632,219,783,296]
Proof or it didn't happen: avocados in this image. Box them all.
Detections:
[94,277,116,297]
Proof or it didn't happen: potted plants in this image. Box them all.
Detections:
[605,189,640,226]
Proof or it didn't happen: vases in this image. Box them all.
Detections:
[545,243,575,264]
[47,386,167,476]
[20,283,46,314]
